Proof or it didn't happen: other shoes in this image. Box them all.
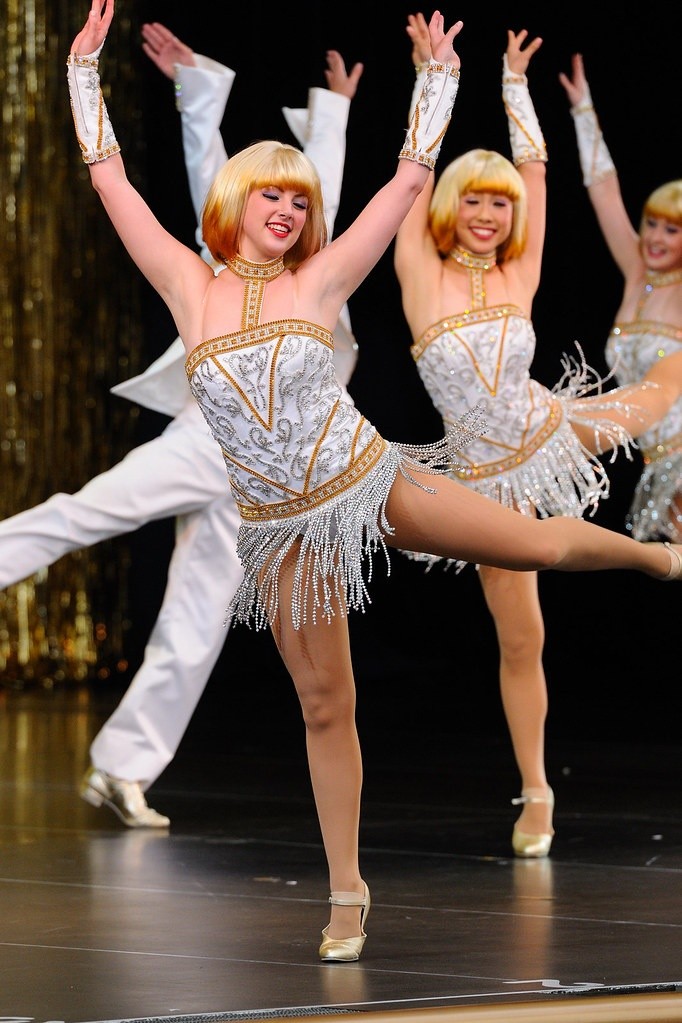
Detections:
[511,784,555,857]
[318,877,370,961]
[78,765,171,827]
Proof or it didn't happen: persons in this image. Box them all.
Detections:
[394,9,682,857]
[555,52,682,545]
[0,22,365,828]
[67,0,682,962]
